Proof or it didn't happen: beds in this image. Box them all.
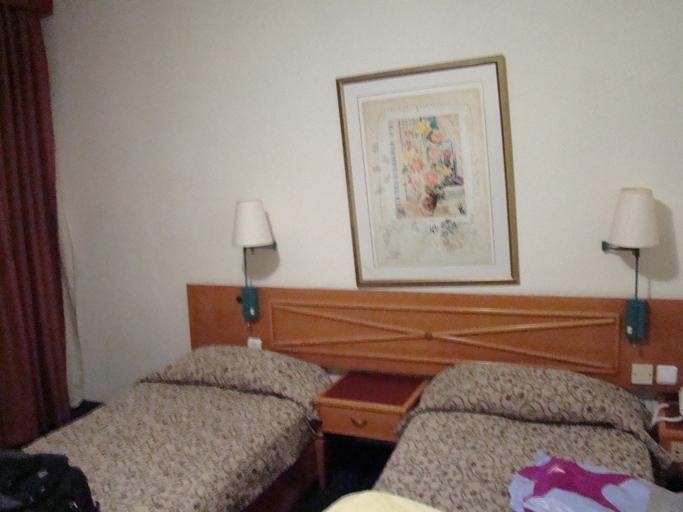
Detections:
[343,361,656,512]
[1,342,343,512]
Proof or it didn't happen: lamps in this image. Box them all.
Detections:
[234,198,274,321]
[603,190,658,345]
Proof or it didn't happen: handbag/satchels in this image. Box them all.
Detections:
[0,448,100,512]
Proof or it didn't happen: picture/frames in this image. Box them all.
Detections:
[336,55,520,287]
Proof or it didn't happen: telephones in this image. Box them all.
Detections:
[678,386,683,415]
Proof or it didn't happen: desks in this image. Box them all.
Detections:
[654,394,683,474]
[311,370,426,478]
